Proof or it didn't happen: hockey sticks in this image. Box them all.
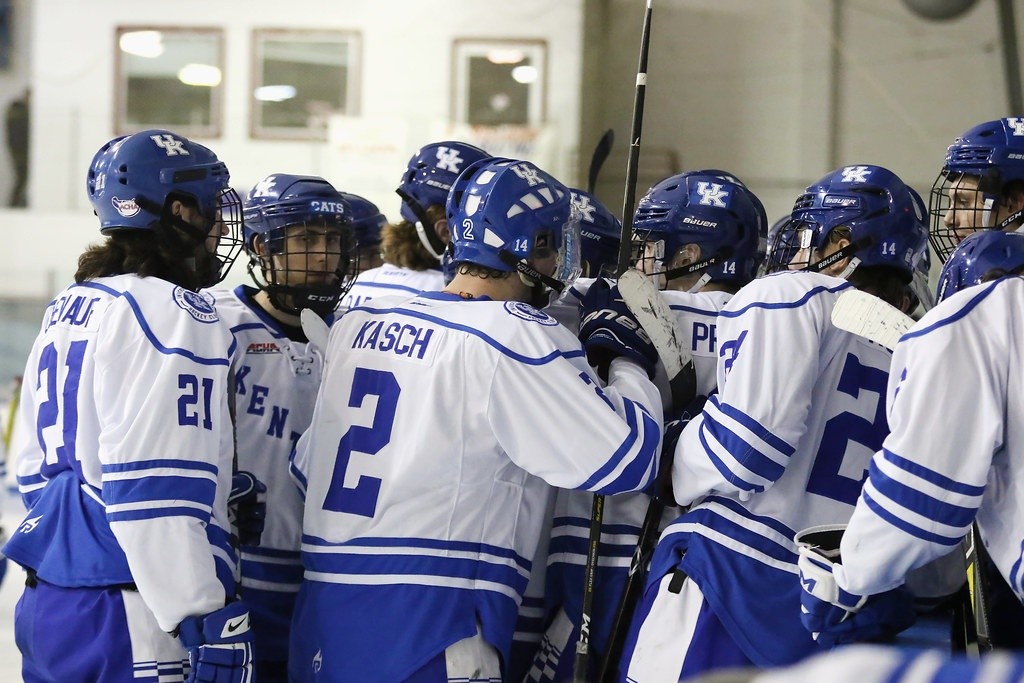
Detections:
[597,267,698,682]
[570,0,655,683]
[829,287,996,659]
[587,128,615,193]
[299,307,332,355]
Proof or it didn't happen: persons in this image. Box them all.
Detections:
[6,84,30,208]
[617,115,931,683]
[0,129,256,683]
[198,174,391,683]
[0,414,8,586]
[679,645,1024,683]
[332,141,492,322]
[508,170,769,683]
[289,156,664,683]
[928,117,1024,661]
[793,229,1024,648]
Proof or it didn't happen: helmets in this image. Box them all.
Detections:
[245,172,360,319]
[567,186,620,272]
[85,129,243,286]
[935,229,1024,305]
[340,190,391,245]
[764,164,929,314]
[397,141,488,258]
[440,157,570,283]
[926,117,1024,262]
[634,169,766,293]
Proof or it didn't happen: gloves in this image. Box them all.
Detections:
[225,468,266,526]
[577,281,657,380]
[179,599,252,683]
[793,521,896,648]
[641,416,689,509]
